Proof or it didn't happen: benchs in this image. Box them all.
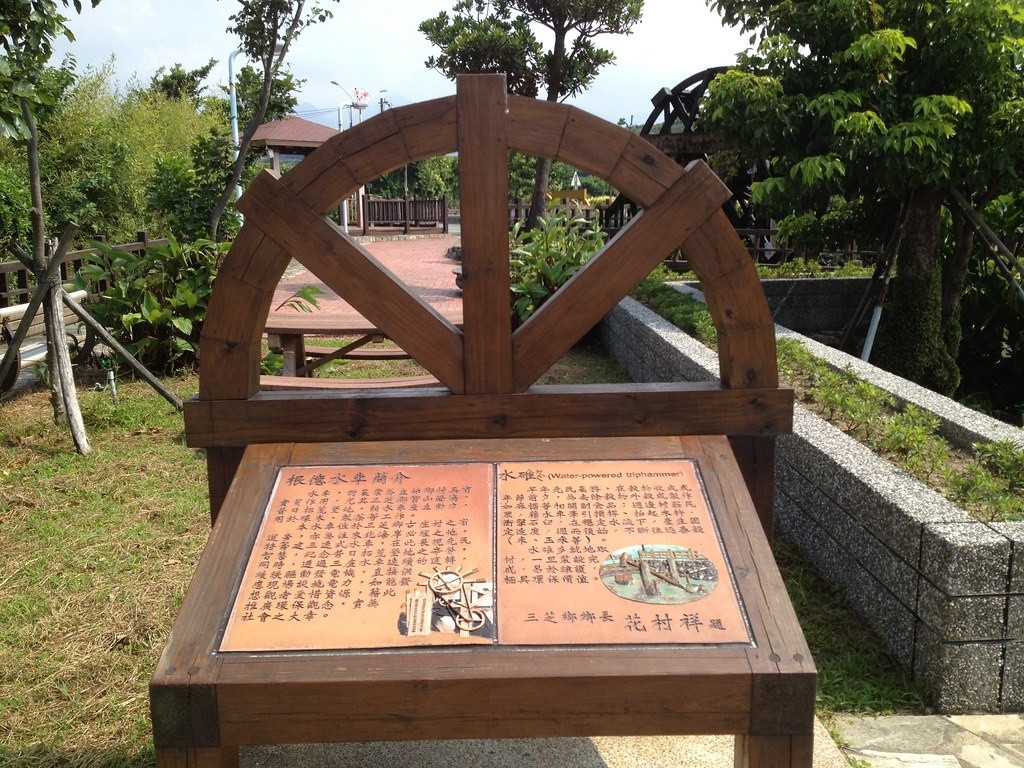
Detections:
[259,373,446,392]
[273,345,413,376]
[0,289,95,394]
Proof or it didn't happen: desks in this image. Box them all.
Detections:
[263,308,464,377]
